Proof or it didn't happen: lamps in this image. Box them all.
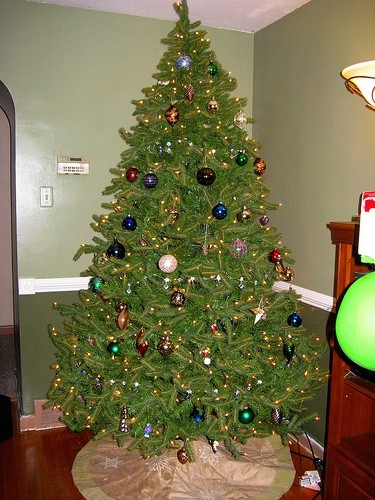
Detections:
[343,60,375,112]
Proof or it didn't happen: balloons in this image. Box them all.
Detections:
[334,270,375,372]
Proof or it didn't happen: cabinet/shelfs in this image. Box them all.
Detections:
[321,216,374,499]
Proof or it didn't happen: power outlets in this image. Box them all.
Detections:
[41,187,52,207]
